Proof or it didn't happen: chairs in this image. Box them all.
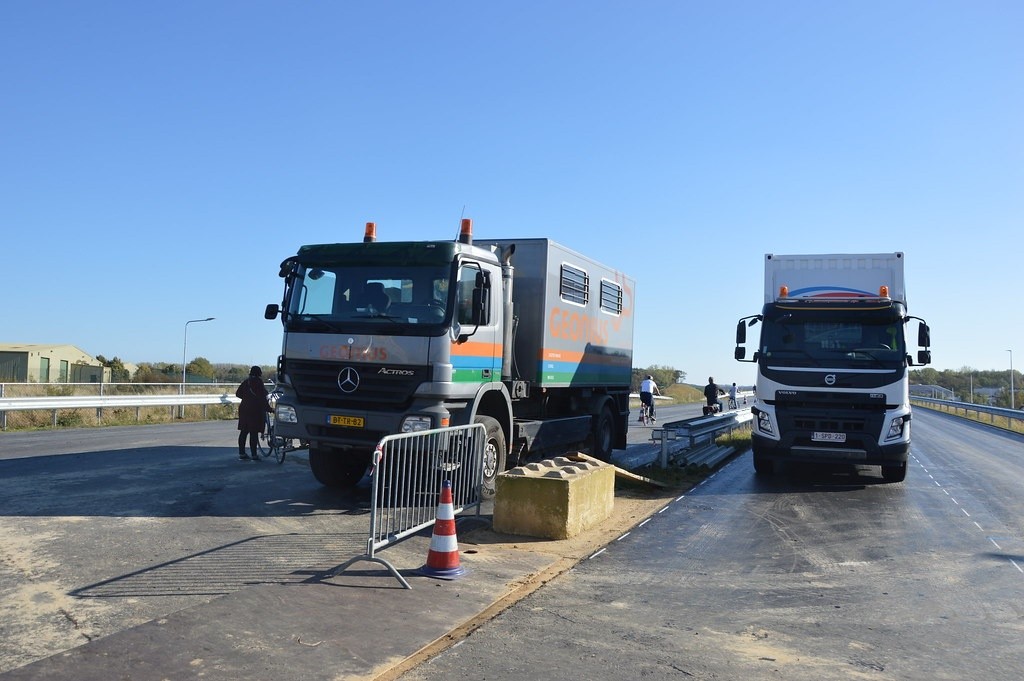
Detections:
[357,282,391,310]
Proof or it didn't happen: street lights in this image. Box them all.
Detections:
[1006,349,1015,410]
[179,317,216,417]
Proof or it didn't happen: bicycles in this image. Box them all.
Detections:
[641,392,662,427]
[728,391,740,410]
[257,386,288,464]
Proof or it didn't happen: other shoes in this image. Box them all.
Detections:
[239,454,251,460]
[650,415,656,421]
[251,457,261,461]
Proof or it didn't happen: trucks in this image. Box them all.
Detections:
[734,250,934,482]
[265,216,637,504]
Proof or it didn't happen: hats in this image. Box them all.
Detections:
[249,366,262,376]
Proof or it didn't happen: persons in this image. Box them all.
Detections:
[235,366,275,463]
[704,377,725,412]
[752,384,757,393]
[640,375,661,421]
[728,383,741,409]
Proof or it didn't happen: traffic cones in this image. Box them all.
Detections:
[412,480,469,581]
[637,402,644,423]
[743,395,747,405]
